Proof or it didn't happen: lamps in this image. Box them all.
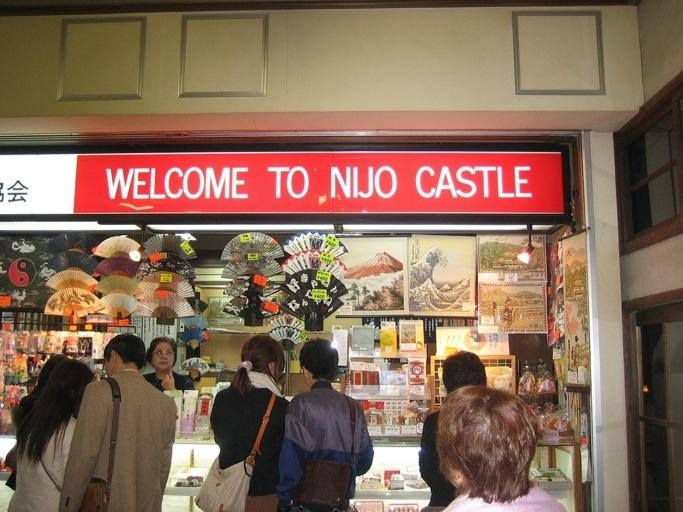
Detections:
[516,224,536,264]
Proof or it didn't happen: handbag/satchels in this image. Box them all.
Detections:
[196,457,256,511]
[79,477,111,511]
[293,460,354,510]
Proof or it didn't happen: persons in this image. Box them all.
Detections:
[3,355,67,490]
[208,334,290,511]
[417,349,487,511]
[57,333,177,511]
[142,336,194,392]
[274,337,374,511]
[434,384,568,512]
[6,358,95,511]
[393,366,406,385]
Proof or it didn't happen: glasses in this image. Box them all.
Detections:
[154,351,175,356]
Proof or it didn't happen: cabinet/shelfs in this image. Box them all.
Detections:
[164,433,220,497]
[349,435,584,512]
